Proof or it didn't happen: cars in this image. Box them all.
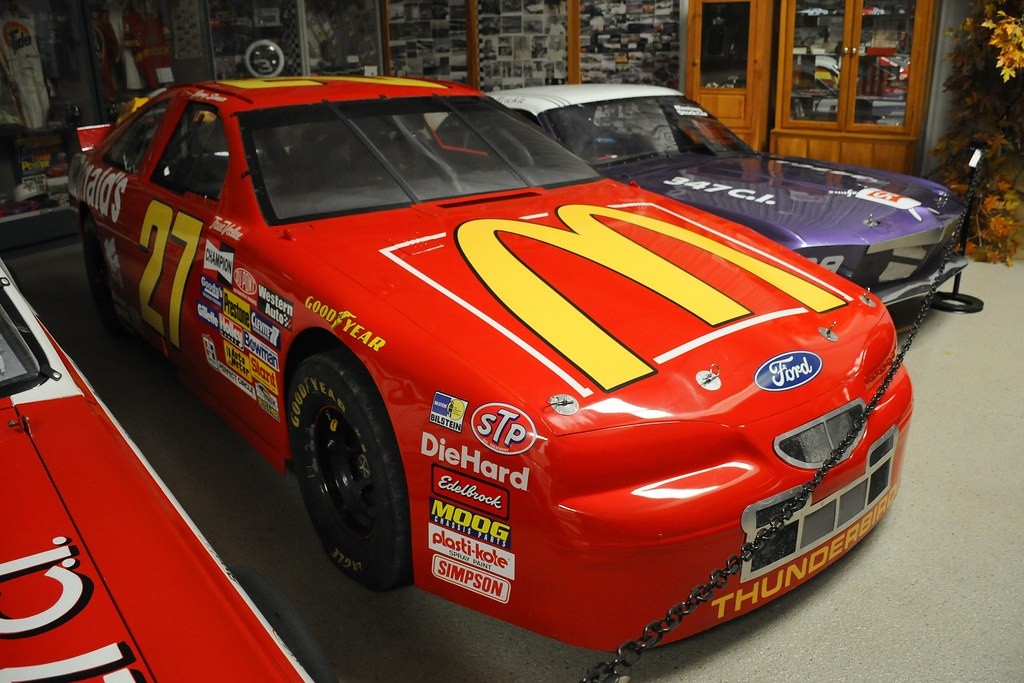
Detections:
[64,73,913,655]
[421,83,966,332]
[0,259,315,683]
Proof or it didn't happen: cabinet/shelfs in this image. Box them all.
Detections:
[686,0,942,179]
